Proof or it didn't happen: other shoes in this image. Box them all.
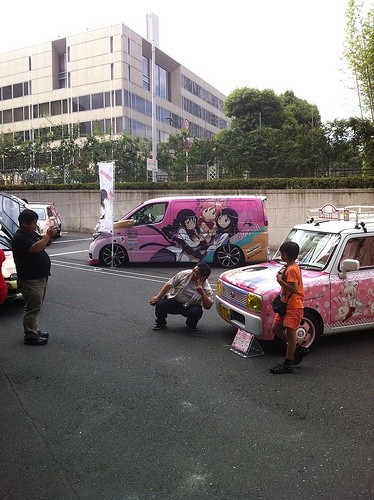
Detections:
[24,330,50,345]
[187,325,199,331]
[152,318,167,330]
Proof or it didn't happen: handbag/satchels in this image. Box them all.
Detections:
[271,295,287,316]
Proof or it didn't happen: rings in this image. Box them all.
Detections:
[152,302,155,304]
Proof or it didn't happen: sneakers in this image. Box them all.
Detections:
[269,345,307,374]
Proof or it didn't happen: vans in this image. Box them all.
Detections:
[87,195,268,268]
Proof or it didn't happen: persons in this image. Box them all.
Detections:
[150,263,214,331]
[10,209,57,345]
[269,242,310,373]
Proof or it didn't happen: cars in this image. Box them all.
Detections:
[0,192,39,305]
[215,205,374,351]
[29,205,62,240]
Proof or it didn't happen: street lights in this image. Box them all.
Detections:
[167,116,182,130]
